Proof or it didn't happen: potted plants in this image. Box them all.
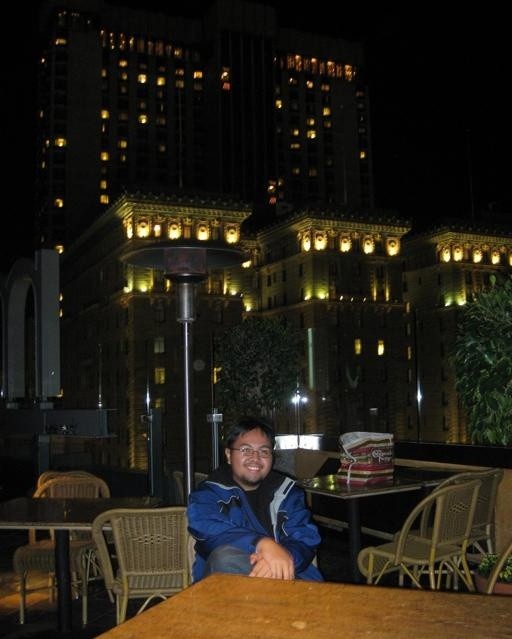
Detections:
[473,548,511,595]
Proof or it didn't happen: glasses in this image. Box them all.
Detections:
[229,445,273,457]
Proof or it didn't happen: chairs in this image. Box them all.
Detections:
[90,505,200,624]
[409,468,507,581]
[355,482,482,589]
[12,474,113,621]
[36,470,123,602]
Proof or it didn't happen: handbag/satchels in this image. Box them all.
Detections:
[336,431,395,488]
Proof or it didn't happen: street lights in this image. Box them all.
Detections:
[119,238,244,507]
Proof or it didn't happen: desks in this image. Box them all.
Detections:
[295,472,456,570]
[91,570,512,639]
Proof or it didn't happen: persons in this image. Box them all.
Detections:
[186,416,327,584]
